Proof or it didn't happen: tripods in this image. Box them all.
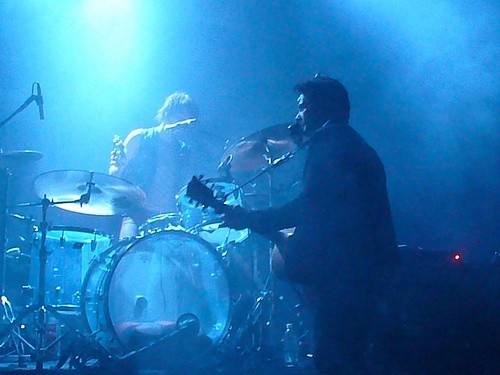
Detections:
[0,183,92,370]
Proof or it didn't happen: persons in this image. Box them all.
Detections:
[111,91,203,240]
[223,75,413,375]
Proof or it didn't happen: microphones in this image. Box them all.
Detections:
[216,155,231,172]
[180,319,197,329]
[36,82,44,120]
[164,118,197,134]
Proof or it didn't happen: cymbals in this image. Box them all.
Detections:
[32,169,147,215]
[218,123,295,173]
[1,150,43,170]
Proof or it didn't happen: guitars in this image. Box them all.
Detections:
[184,176,297,282]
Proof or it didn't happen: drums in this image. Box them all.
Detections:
[27,225,113,325]
[137,213,180,235]
[79,229,233,375]
[175,177,249,246]
